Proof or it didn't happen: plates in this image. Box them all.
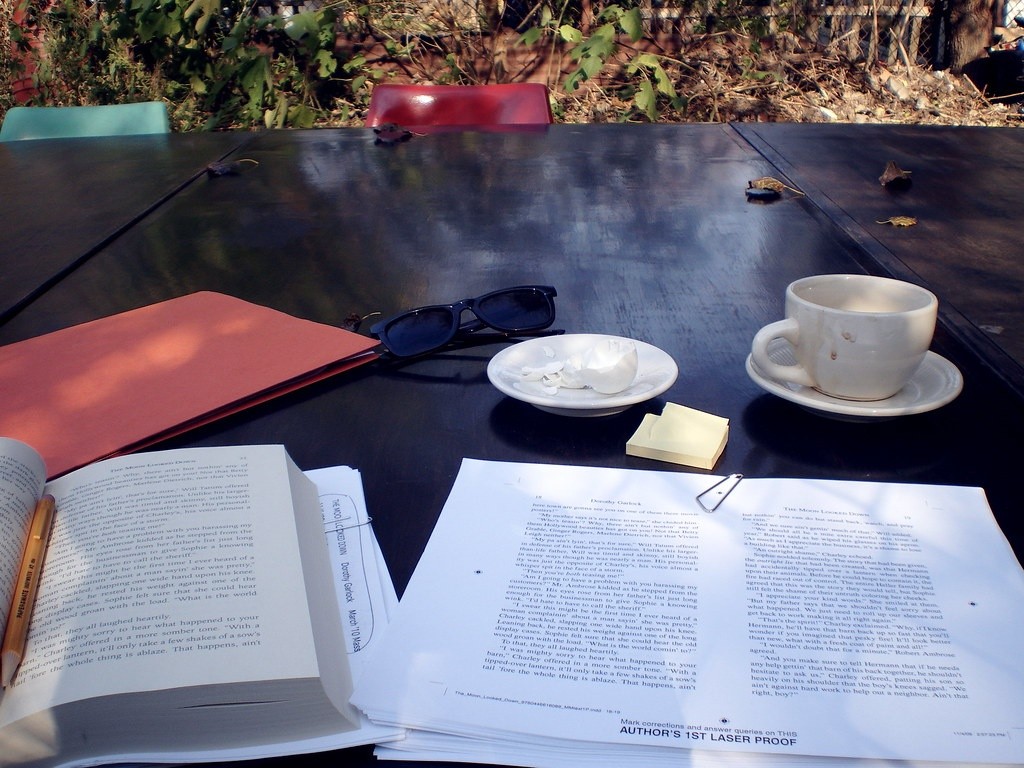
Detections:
[486,333,679,416]
[746,338,962,421]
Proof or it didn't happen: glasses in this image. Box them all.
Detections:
[370,284,565,363]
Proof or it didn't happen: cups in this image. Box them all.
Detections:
[752,275,938,400]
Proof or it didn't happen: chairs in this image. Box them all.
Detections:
[0,100,169,145]
[364,83,554,127]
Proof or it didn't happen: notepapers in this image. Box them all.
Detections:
[625,401,729,471]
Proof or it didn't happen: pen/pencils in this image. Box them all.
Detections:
[0,494,57,688]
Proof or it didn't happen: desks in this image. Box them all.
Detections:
[0,121,1024,767]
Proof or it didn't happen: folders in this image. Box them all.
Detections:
[0,291,384,483]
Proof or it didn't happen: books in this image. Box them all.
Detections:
[0,435,361,768]
